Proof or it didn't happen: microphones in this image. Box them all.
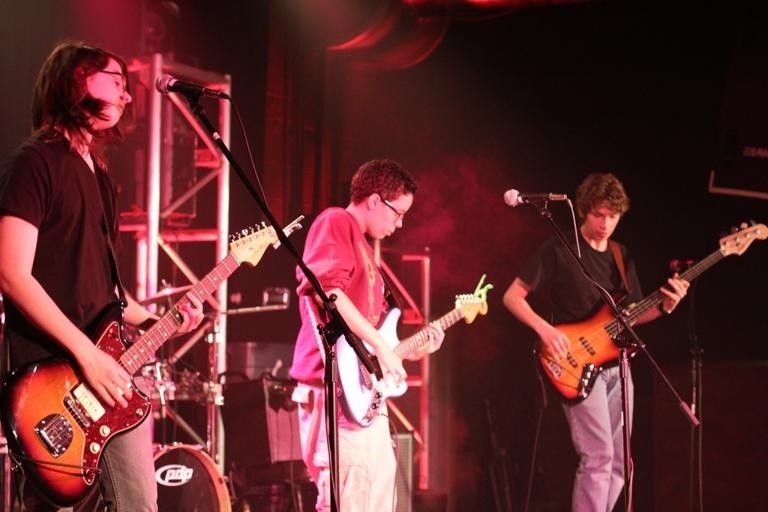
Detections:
[156,72,229,102]
[504,188,567,207]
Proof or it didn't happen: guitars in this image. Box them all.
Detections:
[334,294,488,429]
[1,222,281,508]
[531,219,768,403]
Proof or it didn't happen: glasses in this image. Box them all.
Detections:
[385,200,405,219]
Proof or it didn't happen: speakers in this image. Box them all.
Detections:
[638,353,768,511]
[388,431,414,512]
[220,374,303,467]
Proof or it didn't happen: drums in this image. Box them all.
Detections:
[151,444,232,511]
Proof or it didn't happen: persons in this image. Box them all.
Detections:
[0,40,205,511]
[503,170,690,512]
[285,156,447,512]
